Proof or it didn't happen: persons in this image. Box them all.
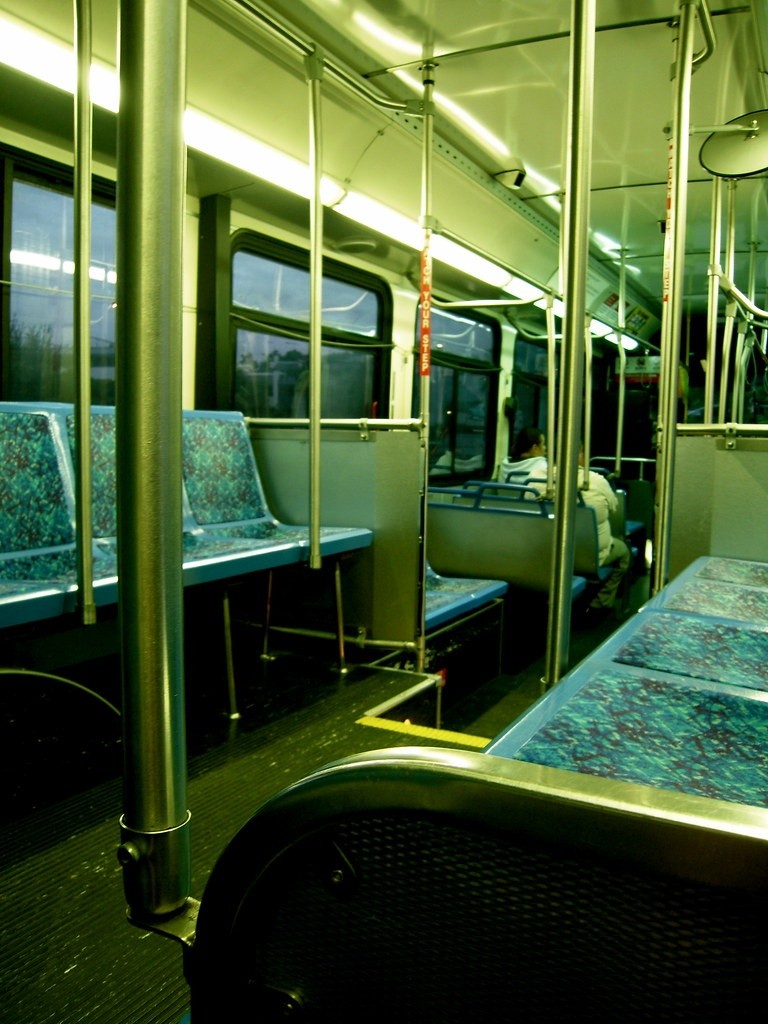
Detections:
[525,435,630,614]
[498,428,548,501]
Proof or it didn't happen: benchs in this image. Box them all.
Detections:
[0,401,767,1024]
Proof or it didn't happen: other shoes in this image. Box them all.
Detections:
[585,606,616,630]
